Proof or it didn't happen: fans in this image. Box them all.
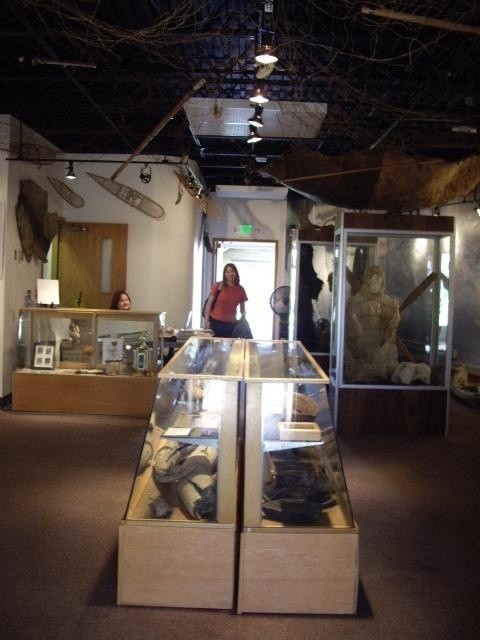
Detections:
[270,287,292,337]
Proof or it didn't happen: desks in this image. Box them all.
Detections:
[160,339,186,356]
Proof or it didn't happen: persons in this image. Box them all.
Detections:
[204,262,249,338]
[109,290,132,310]
[345,263,400,380]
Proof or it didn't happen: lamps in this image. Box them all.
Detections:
[37,279,61,305]
[66,160,77,180]
[247,30,278,144]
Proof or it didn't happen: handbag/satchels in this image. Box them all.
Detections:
[202,282,223,321]
[232,310,253,339]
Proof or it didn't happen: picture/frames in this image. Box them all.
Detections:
[32,341,55,370]
